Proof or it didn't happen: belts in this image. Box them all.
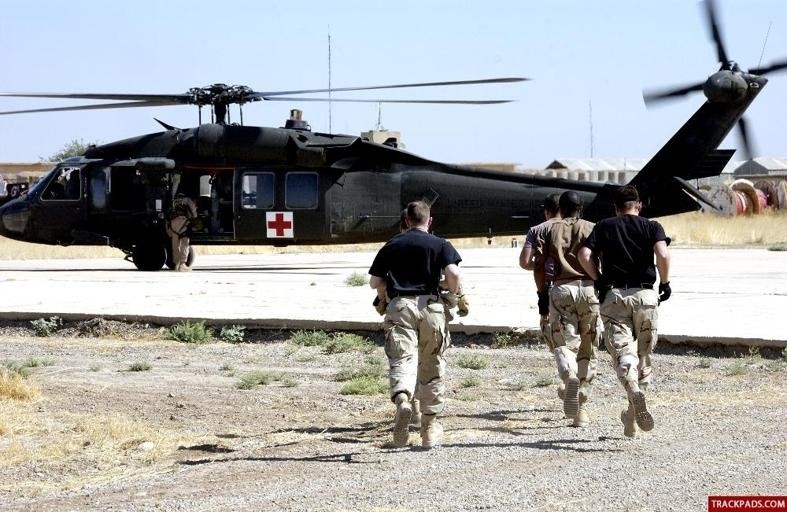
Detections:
[613,283,653,289]
[395,290,438,297]
[568,277,592,280]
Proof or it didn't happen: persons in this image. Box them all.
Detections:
[371,198,459,451]
[375,207,473,425]
[518,193,602,403]
[534,190,604,427]
[575,184,671,437]
[162,192,197,273]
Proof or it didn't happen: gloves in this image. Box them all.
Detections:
[659,283,672,301]
[442,293,469,317]
[592,275,608,303]
[537,292,549,315]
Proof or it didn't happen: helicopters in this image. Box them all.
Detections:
[1,0,787,270]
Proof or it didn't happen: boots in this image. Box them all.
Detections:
[175,257,189,271]
[559,370,588,426]
[621,381,654,437]
[393,393,443,448]
